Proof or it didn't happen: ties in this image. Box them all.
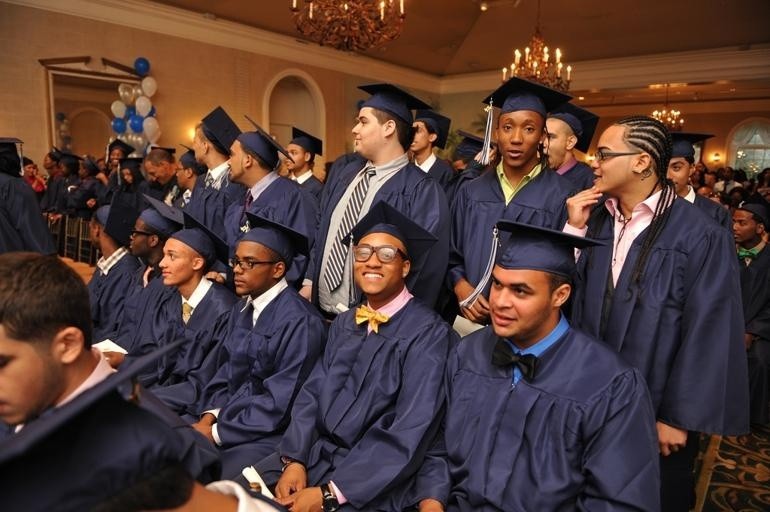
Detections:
[182,303,191,324]
[323,167,376,293]
[240,191,253,224]
[143,266,153,289]
[206,173,214,189]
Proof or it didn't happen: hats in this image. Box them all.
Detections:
[117,158,144,185]
[201,106,242,154]
[83,157,100,176]
[171,211,229,267]
[291,126,322,156]
[151,146,175,154]
[415,108,451,150]
[454,130,497,161]
[0,137,24,176]
[237,115,295,170]
[60,153,85,171]
[356,82,433,125]
[105,138,136,163]
[2,339,190,510]
[96,192,138,248]
[23,157,33,166]
[547,103,600,154]
[140,193,185,237]
[240,211,310,269]
[49,145,64,165]
[670,133,716,157]
[179,144,208,175]
[481,76,574,119]
[734,192,769,229]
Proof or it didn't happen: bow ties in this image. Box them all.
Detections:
[739,247,758,259]
[355,305,389,334]
[492,338,537,383]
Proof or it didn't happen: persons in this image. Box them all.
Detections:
[734,201,770,442]
[191,105,246,246]
[449,78,583,344]
[140,212,239,424]
[1,137,56,253]
[540,102,600,188]
[453,128,499,193]
[401,220,662,510]
[146,150,178,200]
[1,339,292,511]
[116,194,185,355]
[235,200,454,511]
[62,153,79,204]
[41,149,62,239]
[410,109,460,195]
[299,83,451,325]
[563,116,752,511]
[71,160,106,241]
[173,144,208,207]
[1,252,223,510]
[285,126,323,203]
[85,190,142,346]
[198,211,330,481]
[666,133,733,237]
[118,157,143,198]
[693,160,769,209]
[106,139,136,176]
[224,114,308,284]
[20,156,47,191]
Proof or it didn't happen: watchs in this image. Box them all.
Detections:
[320,482,340,512]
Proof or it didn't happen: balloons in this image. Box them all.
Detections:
[142,117,159,141]
[126,106,136,120]
[130,116,145,134]
[137,97,152,119]
[111,100,126,118]
[108,133,148,151]
[120,84,132,106]
[112,120,125,135]
[134,85,143,100]
[135,57,149,76]
[143,78,157,98]
[111,119,126,131]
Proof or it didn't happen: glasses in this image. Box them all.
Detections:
[130,226,153,237]
[226,256,278,272]
[594,150,641,161]
[351,243,411,265]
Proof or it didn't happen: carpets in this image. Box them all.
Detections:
[689,428,770,512]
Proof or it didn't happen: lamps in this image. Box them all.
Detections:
[502,30,573,95]
[649,108,685,132]
[287,0,407,54]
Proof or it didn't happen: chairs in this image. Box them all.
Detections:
[77,217,94,263]
[47,211,61,252]
[65,215,77,259]
